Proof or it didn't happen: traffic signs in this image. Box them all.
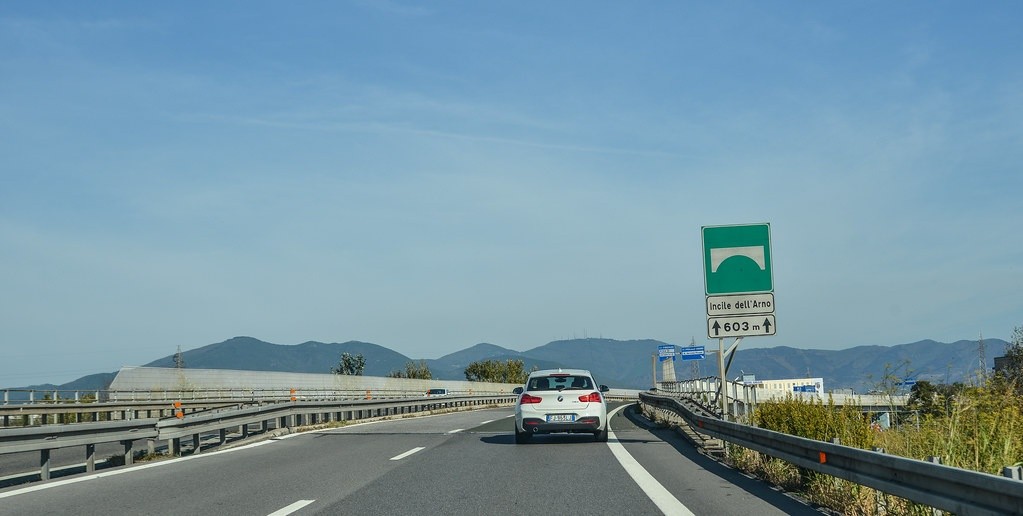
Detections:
[705,293,778,337]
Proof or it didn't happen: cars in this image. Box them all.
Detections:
[512,368,610,442]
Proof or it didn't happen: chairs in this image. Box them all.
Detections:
[536,378,549,389]
[570,377,585,389]
